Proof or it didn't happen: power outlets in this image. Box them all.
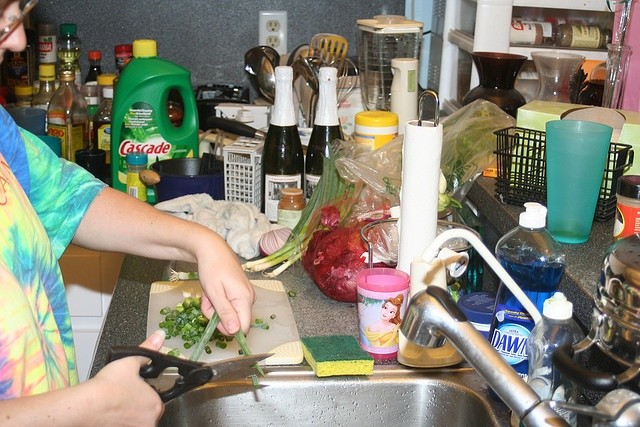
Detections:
[259,10,288,56]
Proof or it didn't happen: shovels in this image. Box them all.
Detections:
[308,33,348,78]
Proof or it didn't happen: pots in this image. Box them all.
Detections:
[139,158,226,203]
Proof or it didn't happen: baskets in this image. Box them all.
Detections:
[222,136,266,213]
[492,126,634,222]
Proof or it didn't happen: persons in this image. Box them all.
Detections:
[0,0,258,426]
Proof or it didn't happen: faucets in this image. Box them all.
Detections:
[398,286,637,426]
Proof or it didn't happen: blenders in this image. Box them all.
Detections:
[356,14,423,112]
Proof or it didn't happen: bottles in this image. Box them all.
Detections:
[34,20,59,90]
[276,189,307,228]
[47,68,86,164]
[114,44,133,67]
[83,50,101,83]
[556,24,610,48]
[58,22,82,86]
[510,23,543,44]
[512,16,546,24]
[305,65,345,202]
[95,87,111,149]
[458,291,495,339]
[544,16,587,26]
[84,97,99,147]
[96,71,117,100]
[12,85,32,106]
[525,291,586,427]
[82,80,98,148]
[126,151,146,203]
[354,109,399,149]
[260,65,304,223]
[32,65,57,110]
[613,175,640,242]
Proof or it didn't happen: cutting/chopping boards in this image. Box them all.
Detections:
[144,279,305,366]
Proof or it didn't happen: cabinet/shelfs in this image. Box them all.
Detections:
[440,0,612,108]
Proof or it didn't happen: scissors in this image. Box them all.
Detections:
[105,346,275,404]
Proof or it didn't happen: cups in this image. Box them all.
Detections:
[356,267,410,361]
[547,119,613,243]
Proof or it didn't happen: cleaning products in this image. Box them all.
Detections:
[111,39,199,203]
[526,292,578,426]
[488,201,567,373]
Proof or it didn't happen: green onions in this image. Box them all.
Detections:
[168,269,199,282]
[190,311,221,363]
[239,139,355,278]
[234,329,265,378]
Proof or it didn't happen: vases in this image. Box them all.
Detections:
[460,51,528,115]
[531,50,585,103]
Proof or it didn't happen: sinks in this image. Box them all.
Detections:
[132,365,525,427]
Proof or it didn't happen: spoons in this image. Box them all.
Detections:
[257,56,275,103]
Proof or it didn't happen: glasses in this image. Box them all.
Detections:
[0,1,37,43]
